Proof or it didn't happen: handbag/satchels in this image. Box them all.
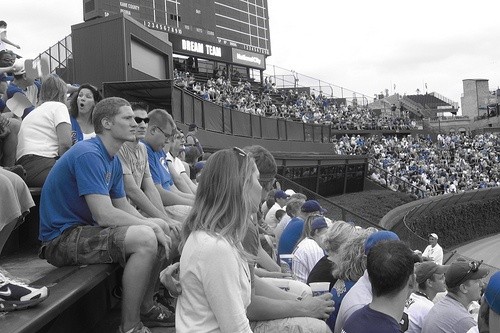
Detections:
[2,163,31,188]
[264,234,278,263]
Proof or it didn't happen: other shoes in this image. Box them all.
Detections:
[112,282,175,313]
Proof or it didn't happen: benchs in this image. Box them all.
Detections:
[0,187,121,333]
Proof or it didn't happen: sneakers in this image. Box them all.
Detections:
[119,322,152,333]
[141,298,175,327]
[0,271,50,311]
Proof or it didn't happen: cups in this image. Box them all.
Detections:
[309,282,331,297]
[279,254,294,273]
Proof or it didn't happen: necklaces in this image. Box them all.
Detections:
[416,290,431,300]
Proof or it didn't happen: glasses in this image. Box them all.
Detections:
[233,146,247,160]
[134,117,150,123]
[455,261,478,284]
[157,127,170,138]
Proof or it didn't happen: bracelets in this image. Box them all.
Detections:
[297,298,302,301]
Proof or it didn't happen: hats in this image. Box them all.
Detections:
[301,200,328,213]
[364,231,401,255]
[189,124,198,131]
[0,73,14,82]
[456,256,466,261]
[12,58,37,75]
[445,259,490,289]
[415,261,450,284]
[310,217,327,229]
[428,233,438,240]
[275,190,290,199]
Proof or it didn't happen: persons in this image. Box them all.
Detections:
[0,22,500,333]
